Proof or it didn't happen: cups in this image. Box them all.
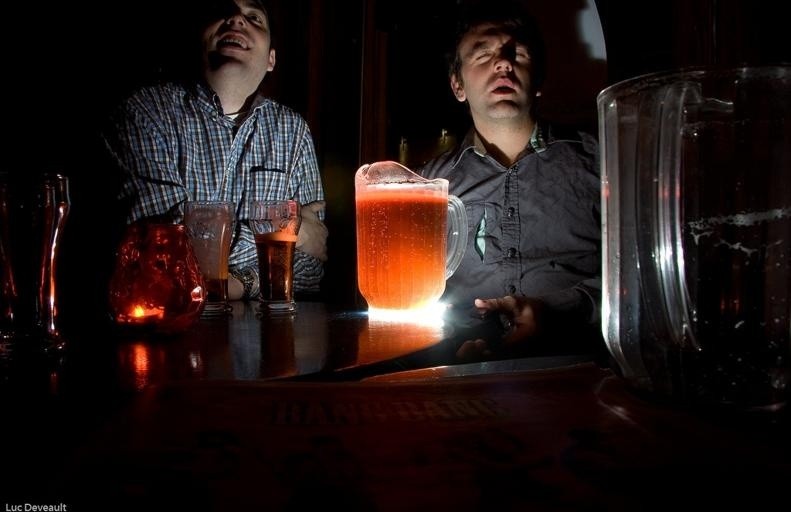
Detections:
[353,158,470,319]
[0,171,73,355]
[102,213,208,333]
[595,62,791,410]
[178,198,235,316]
[243,197,301,315]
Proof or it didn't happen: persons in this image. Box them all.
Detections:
[100,0,331,302]
[406,15,664,374]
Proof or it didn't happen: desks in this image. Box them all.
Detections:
[0,293,446,383]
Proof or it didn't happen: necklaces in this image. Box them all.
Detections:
[221,108,249,122]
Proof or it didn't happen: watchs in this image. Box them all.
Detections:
[228,266,257,303]
